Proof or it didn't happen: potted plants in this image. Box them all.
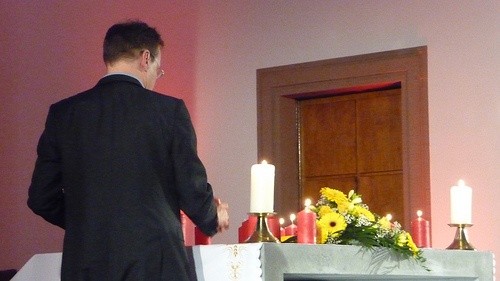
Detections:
[250,164,275,213]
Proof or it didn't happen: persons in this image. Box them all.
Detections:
[26,19,230,281]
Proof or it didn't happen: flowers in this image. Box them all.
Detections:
[315,187,430,271]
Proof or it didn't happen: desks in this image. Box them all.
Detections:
[9,243,496,281]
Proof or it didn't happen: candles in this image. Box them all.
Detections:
[449,186,472,224]
[297,211,315,244]
[412,217,430,248]
[239,215,287,243]
[195,226,211,245]
[285,224,297,236]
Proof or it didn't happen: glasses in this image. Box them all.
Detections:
[148,54,165,82]
